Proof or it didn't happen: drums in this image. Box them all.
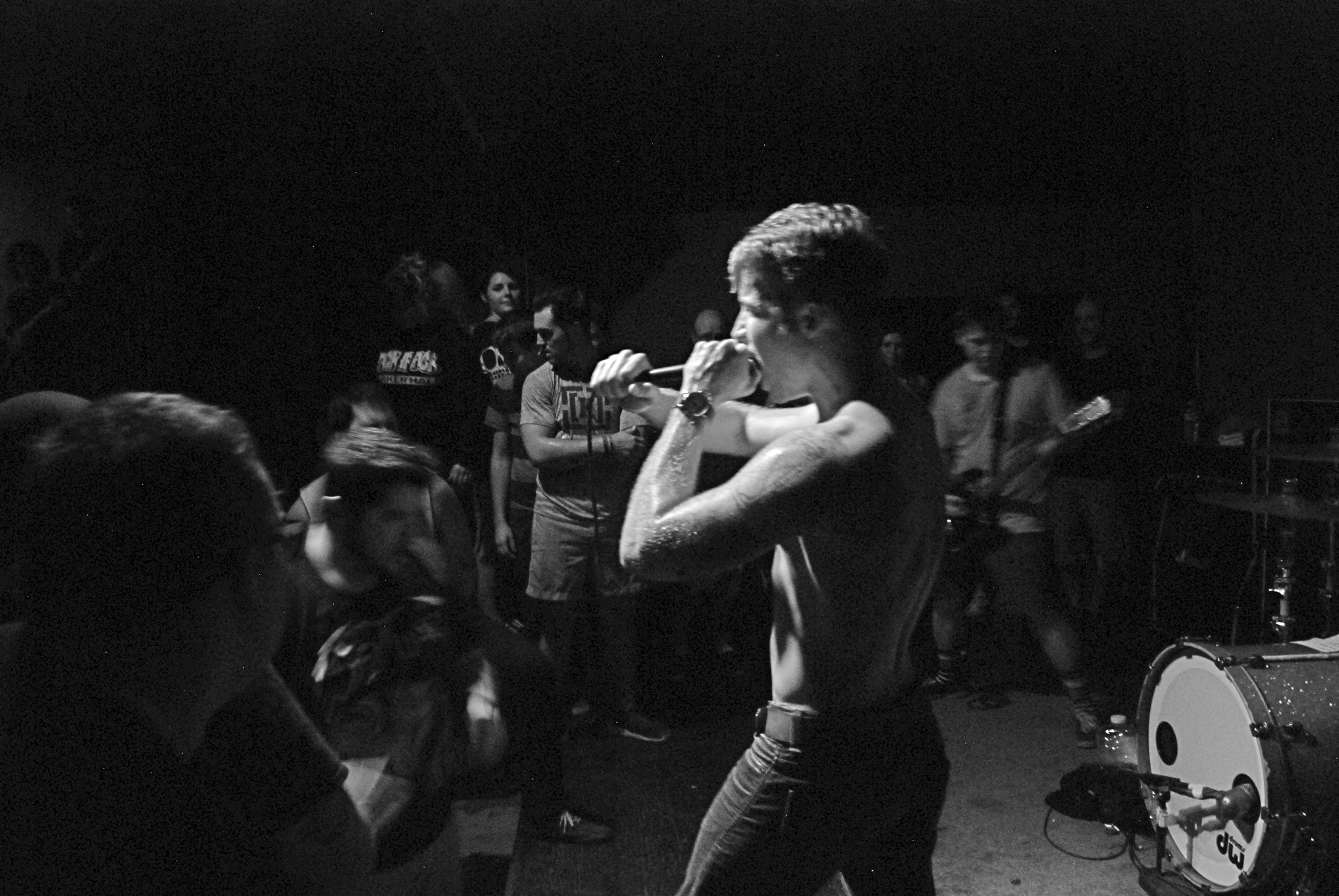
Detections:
[1136,634,1339,894]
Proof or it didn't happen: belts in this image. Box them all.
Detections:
[754,703,931,746]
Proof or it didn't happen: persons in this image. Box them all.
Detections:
[0,193,953,896]
[992,292,1045,365]
[1052,296,1128,627]
[909,302,1106,748]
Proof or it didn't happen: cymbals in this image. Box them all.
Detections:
[1196,491,1339,523]
[1252,436,1339,463]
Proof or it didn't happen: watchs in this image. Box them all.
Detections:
[667,391,715,421]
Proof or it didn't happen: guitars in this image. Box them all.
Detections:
[937,395,1113,574]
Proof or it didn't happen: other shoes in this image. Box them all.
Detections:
[926,668,963,699]
[1073,707,1099,748]
[602,710,671,742]
[536,810,613,842]
[968,687,1007,709]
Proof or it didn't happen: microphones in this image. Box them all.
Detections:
[1216,783,1258,821]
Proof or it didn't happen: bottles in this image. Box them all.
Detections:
[1100,714,1139,768]
